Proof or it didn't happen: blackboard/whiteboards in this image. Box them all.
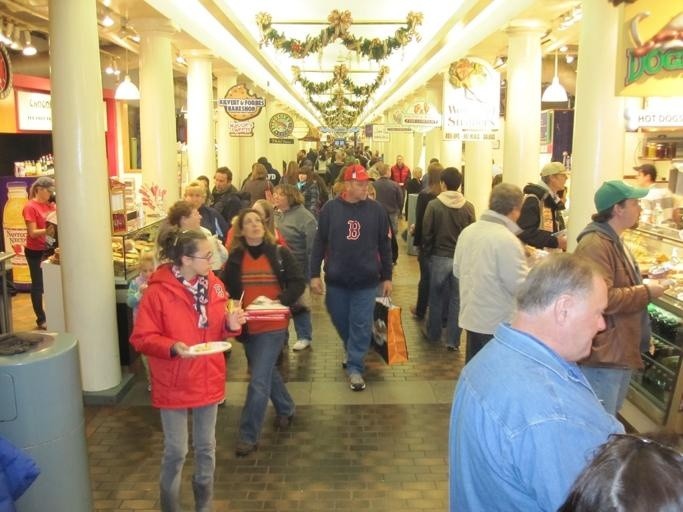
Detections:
[551,108,573,161]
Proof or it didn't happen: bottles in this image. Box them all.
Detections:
[551,228,567,239]
[14,154,54,177]
[630,308,683,406]
[647,142,674,159]
[653,202,663,225]
[1,182,32,285]
[566,155,570,167]
[157,199,164,216]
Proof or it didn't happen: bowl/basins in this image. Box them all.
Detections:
[672,208,682,228]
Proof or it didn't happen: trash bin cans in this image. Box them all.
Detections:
[0,331,97,511]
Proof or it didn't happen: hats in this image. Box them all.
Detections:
[540,161,571,176]
[37,177,56,193]
[594,180,649,211]
[344,165,376,182]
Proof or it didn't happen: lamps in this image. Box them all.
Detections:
[115,17,140,101]
[1,14,37,56]
[541,46,569,103]
[105,55,121,75]
[560,46,574,63]
[557,8,584,28]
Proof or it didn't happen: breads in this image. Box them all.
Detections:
[113,236,154,265]
[623,233,682,301]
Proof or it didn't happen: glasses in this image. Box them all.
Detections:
[608,433,682,458]
[186,250,214,259]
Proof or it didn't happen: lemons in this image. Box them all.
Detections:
[227,298,234,313]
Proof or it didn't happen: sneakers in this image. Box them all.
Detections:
[293,340,311,350]
[235,440,256,454]
[274,401,295,432]
[350,373,365,390]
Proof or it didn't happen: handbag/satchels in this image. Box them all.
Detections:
[371,296,408,364]
[241,309,290,336]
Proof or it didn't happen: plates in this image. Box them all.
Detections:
[184,342,233,355]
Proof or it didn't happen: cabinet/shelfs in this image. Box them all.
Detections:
[619,220,683,433]
[112,212,167,364]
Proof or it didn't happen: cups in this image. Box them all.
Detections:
[225,300,243,331]
[137,207,145,229]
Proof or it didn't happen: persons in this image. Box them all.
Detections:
[126,253,154,392]
[632,163,668,222]
[447,252,627,512]
[128,225,249,512]
[554,428,683,512]
[157,141,571,391]
[217,207,306,456]
[22,176,56,329]
[571,180,671,418]
